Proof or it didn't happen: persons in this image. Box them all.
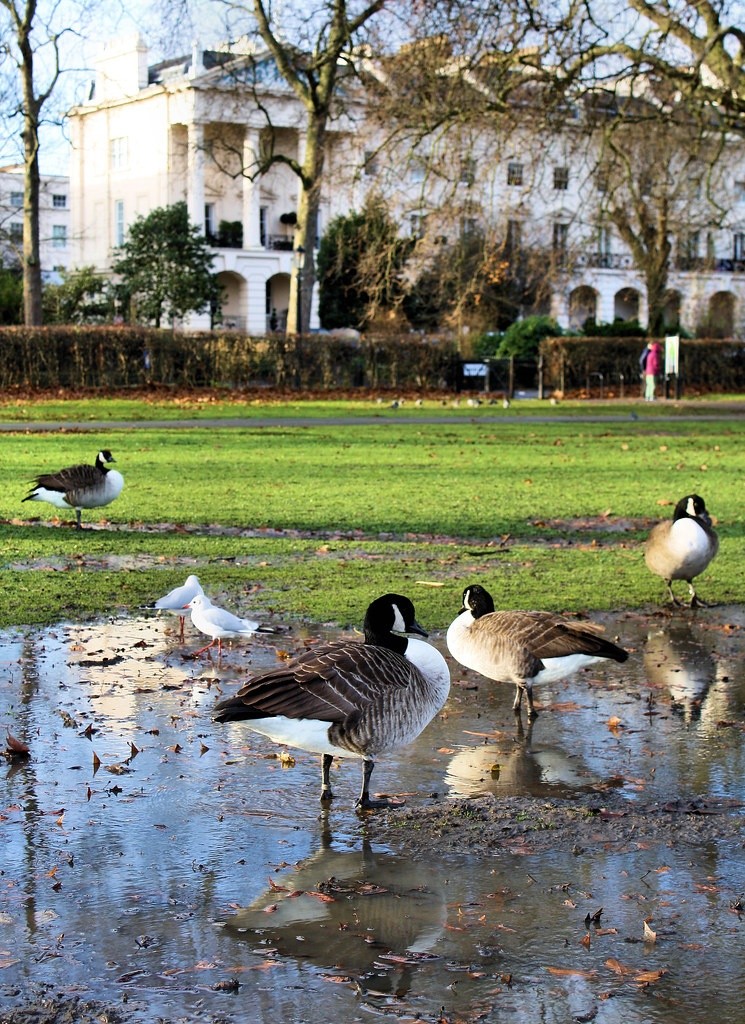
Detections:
[645,341,662,402]
[639,340,657,399]
[272,308,277,311]
[270,313,278,331]
[281,310,286,331]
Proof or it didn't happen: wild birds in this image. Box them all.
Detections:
[643,610,716,722]
[377,396,560,409]
[210,807,447,999]
[442,715,623,799]
[138,575,204,634]
[181,595,275,667]
[21,449,124,529]
[213,594,451,807]
[643,494,718,607]
[447,584,630,725]
